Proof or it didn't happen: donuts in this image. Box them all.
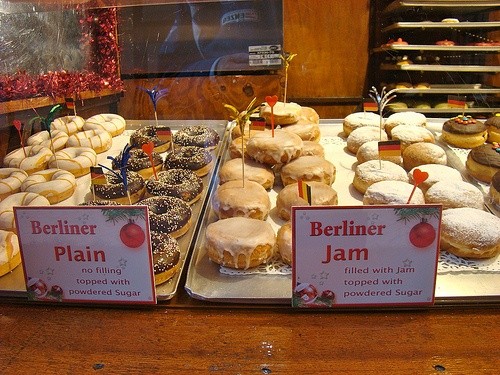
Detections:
[0,114,126,278]
[78,125,222,286]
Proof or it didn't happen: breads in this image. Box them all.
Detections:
[204,102,500,270]
[379,19,500,109]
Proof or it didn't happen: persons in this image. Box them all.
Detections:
[120,0,282,119]
